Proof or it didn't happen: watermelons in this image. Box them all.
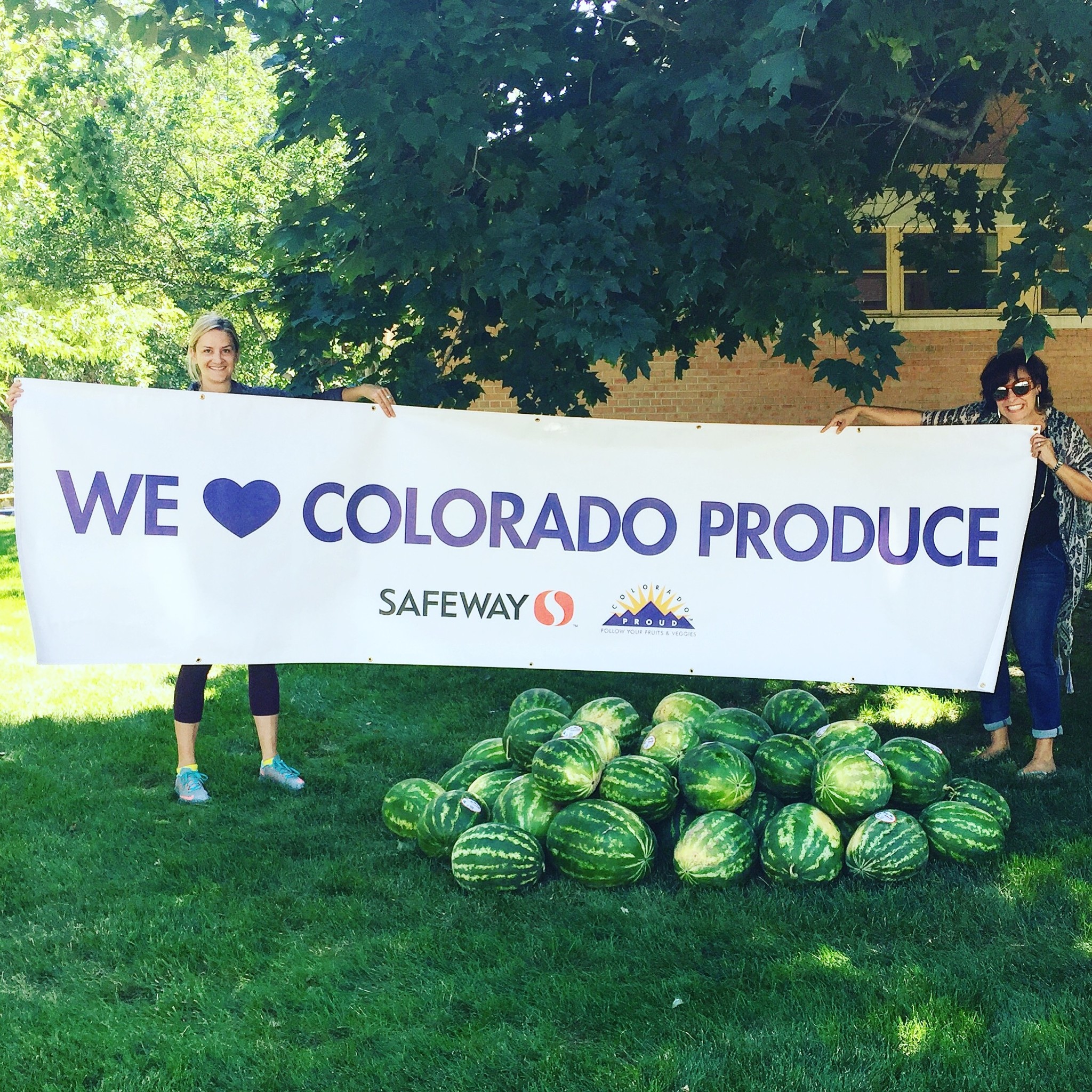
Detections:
[382,688,1011,892]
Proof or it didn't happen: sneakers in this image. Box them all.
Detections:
[174,767,210,802]
[259,754,305,790]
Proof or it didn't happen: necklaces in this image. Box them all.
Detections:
[1029,416,1048,512]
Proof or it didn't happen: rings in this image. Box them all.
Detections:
[386,395,391,399]
[1038,442,1040,446]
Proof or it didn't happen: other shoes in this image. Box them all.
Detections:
[1016,767,1058,780]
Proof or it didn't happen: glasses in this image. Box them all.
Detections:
[992,377,1032,401]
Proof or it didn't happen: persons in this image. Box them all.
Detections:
[5,315,396,803]
[821,346,1092,783]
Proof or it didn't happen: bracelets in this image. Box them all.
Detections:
[1052,461,1063,473]
